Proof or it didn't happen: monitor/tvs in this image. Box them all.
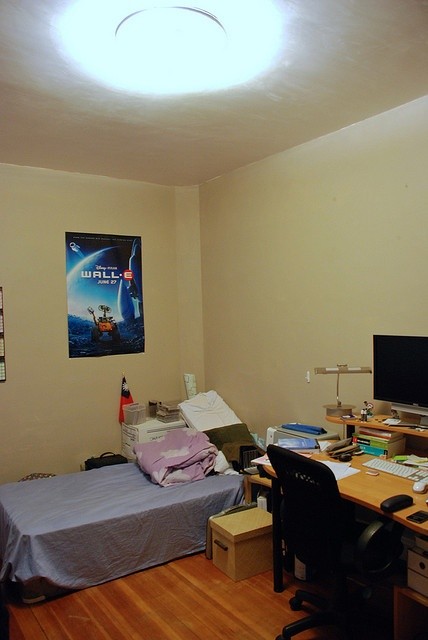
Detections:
[373,335,428,417]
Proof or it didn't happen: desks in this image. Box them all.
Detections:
[252,405,428,640]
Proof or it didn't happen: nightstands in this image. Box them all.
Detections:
[245,444,318,506]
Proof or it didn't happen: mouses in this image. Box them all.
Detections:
[411,484,427,493]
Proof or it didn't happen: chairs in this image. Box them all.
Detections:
[264,443,408,640]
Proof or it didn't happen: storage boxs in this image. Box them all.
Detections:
[120,415,190,460]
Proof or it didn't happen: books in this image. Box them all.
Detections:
[251,453,313,471]
[278,437,320,452]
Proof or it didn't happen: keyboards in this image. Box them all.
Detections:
[363,458,428,483]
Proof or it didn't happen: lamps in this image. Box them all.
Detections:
[312,363,372,422]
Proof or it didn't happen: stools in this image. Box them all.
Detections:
[208,507,278,583]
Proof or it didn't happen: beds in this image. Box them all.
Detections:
[0,440,267,605]
